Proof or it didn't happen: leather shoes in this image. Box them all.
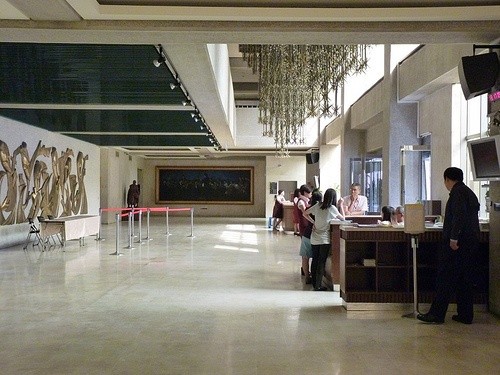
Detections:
[417,312,444,323]
[452,315,473,324]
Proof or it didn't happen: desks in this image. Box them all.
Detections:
[41,215,100,252]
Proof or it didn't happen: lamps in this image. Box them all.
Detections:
[153,59,218,151]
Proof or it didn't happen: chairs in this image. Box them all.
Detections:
[23,218,46,249]
[37,216,45,222]
[48,215,54,220]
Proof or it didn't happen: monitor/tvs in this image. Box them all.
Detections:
[468,135,500,181]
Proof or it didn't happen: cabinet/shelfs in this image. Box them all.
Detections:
[282,204,294,231]
[321,215,383,291]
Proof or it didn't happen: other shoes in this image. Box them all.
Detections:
[274,226,279,231]
[301,267,312,275]
[314,287,328,291]
[306,277,313,284]
[294,232,300,236]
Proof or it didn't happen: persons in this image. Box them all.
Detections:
[302,188,346,291]
[395,205,406,227]
[271,189,290,232]
[414,166,483,326]
[297,183,316,275]
[341,182,369,215]
[379,205,398,226]
[126,179,139,208]
[292,187,301,236]
[296,191,323,285]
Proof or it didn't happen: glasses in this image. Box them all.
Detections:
[349,189,360,191]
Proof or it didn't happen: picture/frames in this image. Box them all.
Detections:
[155,166,253,205]
[270,182,277,194]
